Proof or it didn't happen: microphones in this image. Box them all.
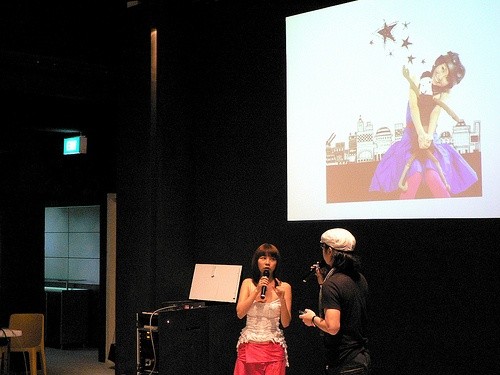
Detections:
[261,270,269,298]
[303,260,326,283]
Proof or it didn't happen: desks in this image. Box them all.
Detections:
[0,328,22,375]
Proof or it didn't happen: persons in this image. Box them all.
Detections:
[234,244,292,375]
[368,51,477,200]
[299,228,373,375]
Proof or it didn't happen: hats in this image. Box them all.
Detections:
[320,228,356,251]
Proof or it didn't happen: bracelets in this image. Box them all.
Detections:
[311,316,318,327]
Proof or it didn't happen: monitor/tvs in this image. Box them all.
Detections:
[189,263,243,303]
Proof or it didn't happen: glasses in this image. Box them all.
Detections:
[320,243,328,249]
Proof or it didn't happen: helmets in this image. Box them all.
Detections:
[430,50,466,92]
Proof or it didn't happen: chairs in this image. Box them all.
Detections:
[0,313,47,375]
